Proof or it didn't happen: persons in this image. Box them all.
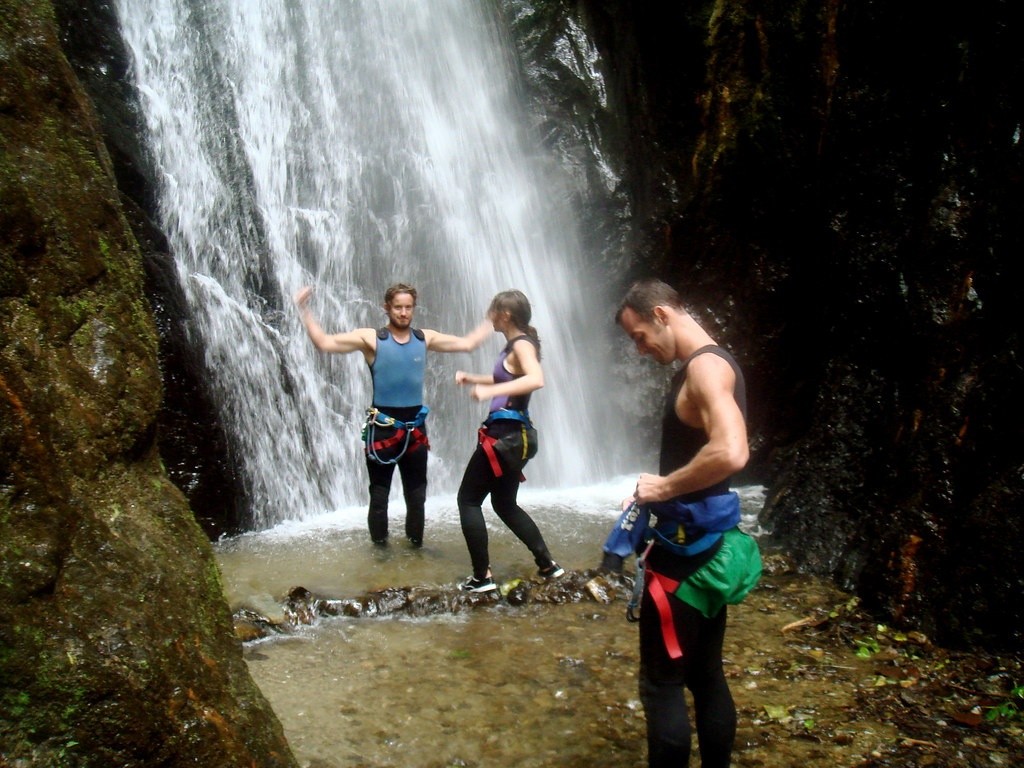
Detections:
[616,280,750,768]
[456,291,565,592]
[296,282,492,545]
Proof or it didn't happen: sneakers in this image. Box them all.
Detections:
[456,574,497,594]
[538,561,565,579]
